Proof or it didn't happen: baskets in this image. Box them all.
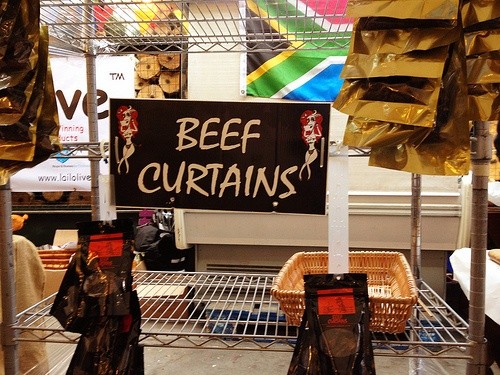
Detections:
[270,251,418,334]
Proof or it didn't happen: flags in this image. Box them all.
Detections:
[239,0,354,102]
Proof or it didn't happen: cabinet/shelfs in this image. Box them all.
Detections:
[0,2,485,375]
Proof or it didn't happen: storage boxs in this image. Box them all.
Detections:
[30,229,81,305]
[133,277,195,322]
